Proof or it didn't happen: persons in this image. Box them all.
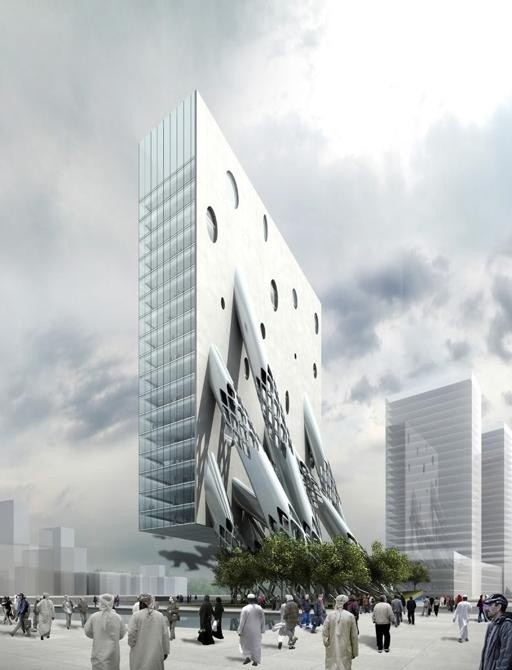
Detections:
[128,593,170,670]
[321,594,359,670]
[83,594,127,670]
[478,594,512,670]
[238,593,264,665]
